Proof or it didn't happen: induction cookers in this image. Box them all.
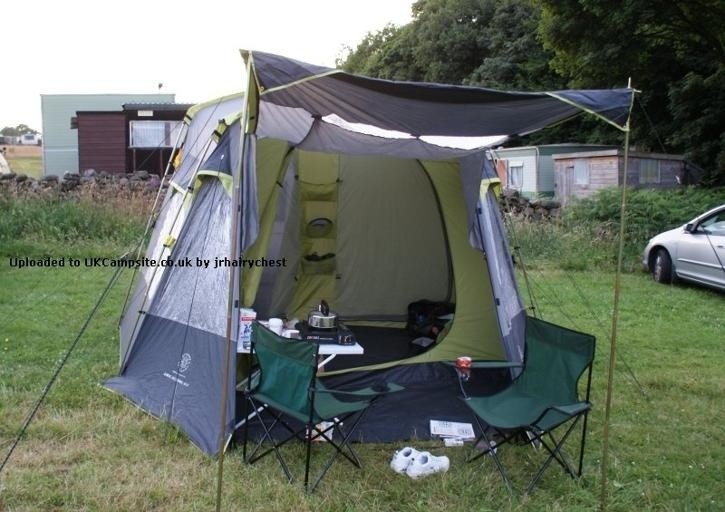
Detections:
[294,318,357,347]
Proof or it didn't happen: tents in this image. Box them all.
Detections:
[94,46,637,470]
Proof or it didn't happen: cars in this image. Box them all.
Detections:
[639,204,724,292]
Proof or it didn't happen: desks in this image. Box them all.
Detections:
[234,321,365,431]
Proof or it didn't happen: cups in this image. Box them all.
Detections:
[269,317,283,336]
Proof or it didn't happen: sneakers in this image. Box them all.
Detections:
[390,447,450,480]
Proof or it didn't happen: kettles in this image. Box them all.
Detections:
[307,301,343,329]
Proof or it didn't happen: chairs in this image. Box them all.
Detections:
[243,320,384,496]
[444,314,596,507]
